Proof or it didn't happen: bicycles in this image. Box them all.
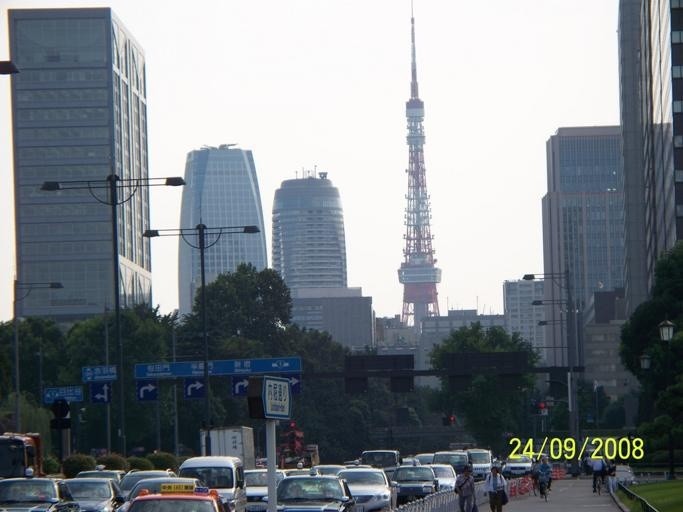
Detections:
[530,471,551,503]
[596,476,602,495]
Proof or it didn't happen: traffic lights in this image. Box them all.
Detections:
[531,402,546,408]
[288,422,296,447]
[447,415,455,423]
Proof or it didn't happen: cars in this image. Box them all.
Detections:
[533,449,606,472]
[616,465,635,483]
[499,455,532,476]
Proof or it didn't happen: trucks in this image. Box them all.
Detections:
[200,425,256,470]
[0,430,44,478]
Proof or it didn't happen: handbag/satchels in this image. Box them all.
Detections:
[502,491,508,505]
[472,504,478,512]
[455,487,461,493]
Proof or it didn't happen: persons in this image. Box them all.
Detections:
[531,459,540,480]
[592,455,607,494]
[454,463,477,512]
[483,466,508,512]
[535,457,552,498]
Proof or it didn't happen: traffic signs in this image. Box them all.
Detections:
[82,365,117,384]
[44,386,83,403]
[134,356,301,378]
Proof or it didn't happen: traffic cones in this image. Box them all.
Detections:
[509,480,517,497]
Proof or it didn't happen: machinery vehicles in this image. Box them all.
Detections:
[277,429,319,468]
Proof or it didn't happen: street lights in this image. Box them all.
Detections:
[522,272,570,297]
[535,318,567,328]
[528,296,570,307]
[40,165,187,467]
[654,309,679,482]
[637,351,653,421]
[141,216,259,457]
[13,277,64,430]
